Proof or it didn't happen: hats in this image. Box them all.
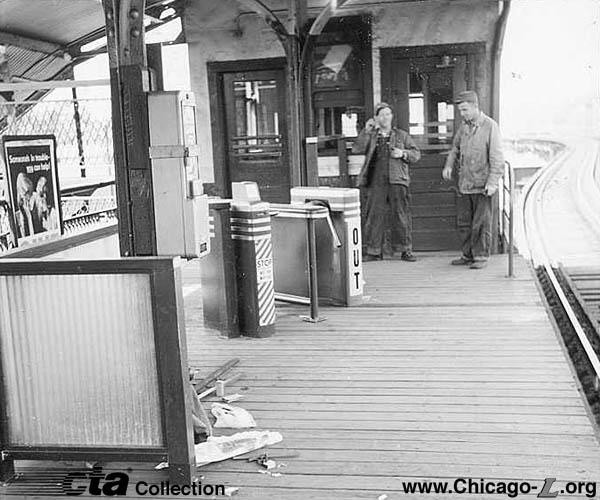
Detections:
[452,91,479,103]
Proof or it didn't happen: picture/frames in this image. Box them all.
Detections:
[2,134,64,250]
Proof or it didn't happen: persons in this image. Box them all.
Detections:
[441,90,505,269]
[352,102,422,263]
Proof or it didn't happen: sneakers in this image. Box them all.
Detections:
[362,254,382,261]
[469,261,487,268]
[453,258,472,264]
[401,253,417,262]
